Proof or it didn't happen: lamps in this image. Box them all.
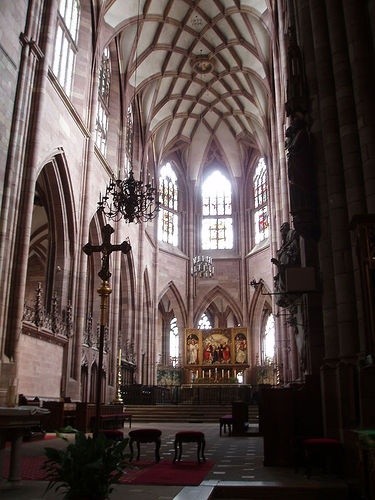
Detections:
[190,254,215,279]
[98,0,163,224]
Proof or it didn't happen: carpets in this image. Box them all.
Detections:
[4,435,60,449]
[1,452,217,486]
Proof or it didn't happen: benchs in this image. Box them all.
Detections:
[99,414,132,430]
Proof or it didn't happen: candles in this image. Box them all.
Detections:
[118,347,123,365]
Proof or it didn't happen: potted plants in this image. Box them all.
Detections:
[38,430,131,500]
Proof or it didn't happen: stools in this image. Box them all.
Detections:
[304,438,347,481]
[219,415,233,438]
[172,431,207,465]
[97,431,125,458]
[129,429,162,462]
